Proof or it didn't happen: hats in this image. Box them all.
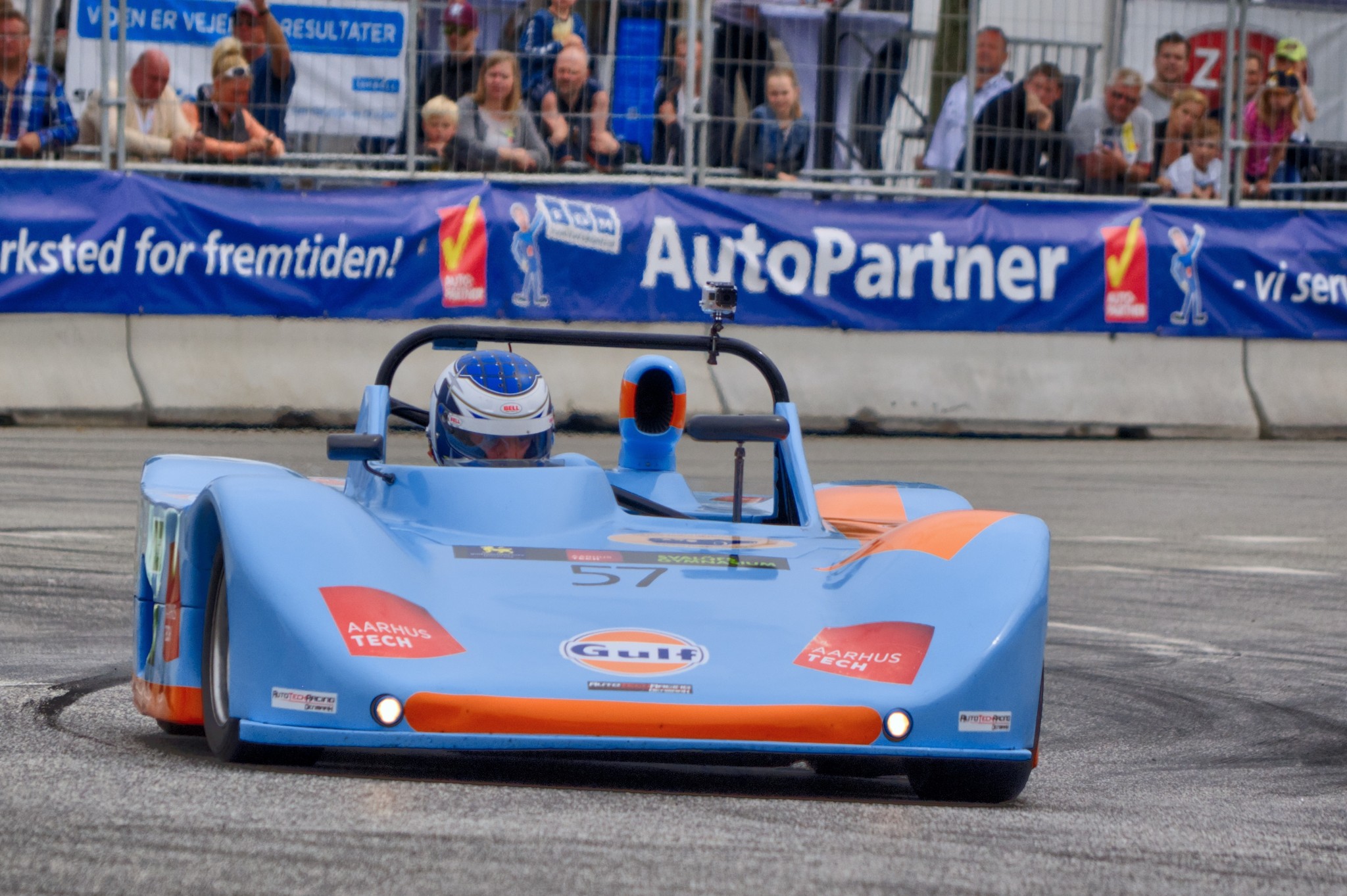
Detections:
[229,1,262,19]
[1274,38,1308,61]
[442,1,479,29]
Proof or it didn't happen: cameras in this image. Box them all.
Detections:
[699,281,738,320]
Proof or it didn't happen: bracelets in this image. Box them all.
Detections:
[258,9,269,17]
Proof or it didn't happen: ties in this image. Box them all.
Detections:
[1103,127,1114,151]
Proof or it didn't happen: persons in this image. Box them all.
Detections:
[229,0,296,189]
[81,48,205,174]
[917,24,1318,204]
[425,349,557,468]
[355,0,641,187]
[0,0,80,161]
[650,0,812,198]
[182,37,286,188]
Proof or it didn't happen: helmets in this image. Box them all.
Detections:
[425,350,557,466]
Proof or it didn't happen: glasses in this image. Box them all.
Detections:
[218,67,247,79]
[231,18,256,29]
[1112,90,1137,104]
[444,22,471,36]
